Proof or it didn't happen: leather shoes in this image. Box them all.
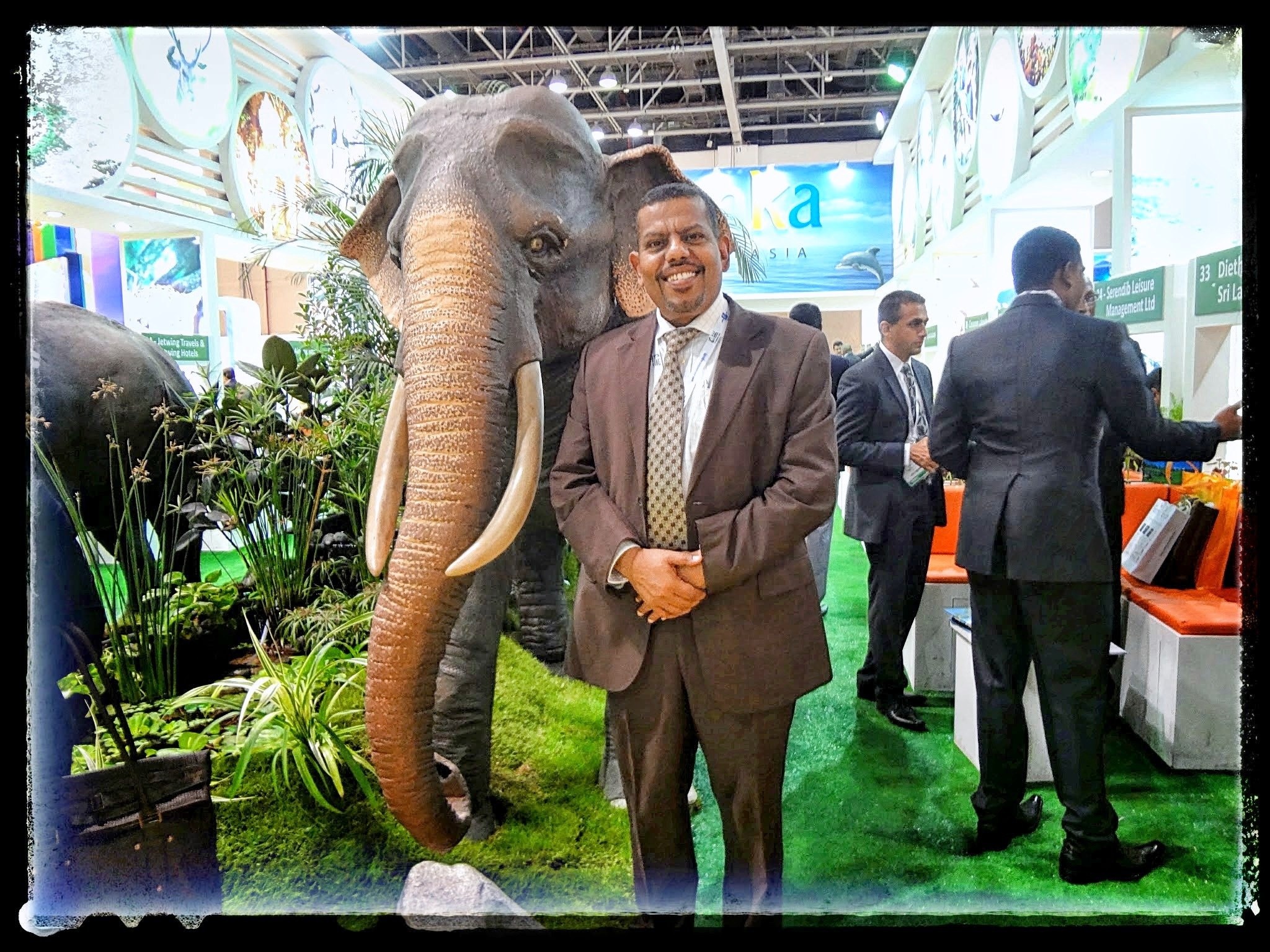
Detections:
[882,703,926,729]
[1058,841,1168,884]
[981,793,1044,850]
[859,686,928,707]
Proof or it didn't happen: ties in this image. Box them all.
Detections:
[900,362,932,485]
[647,327,703,551]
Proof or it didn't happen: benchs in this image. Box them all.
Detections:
[903,485,1242,771]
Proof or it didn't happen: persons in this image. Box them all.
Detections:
[220,368,241,395]
[785,226,1243,884]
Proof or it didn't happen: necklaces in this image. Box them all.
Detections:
[546,182,842,926]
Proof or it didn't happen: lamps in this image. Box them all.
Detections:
[875,64,913,131]
[548,64,643,138]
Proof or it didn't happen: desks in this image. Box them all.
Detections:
[946,609,1126,782]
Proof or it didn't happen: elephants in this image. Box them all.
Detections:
[31,300,209,610]
[335,82,702,852]
